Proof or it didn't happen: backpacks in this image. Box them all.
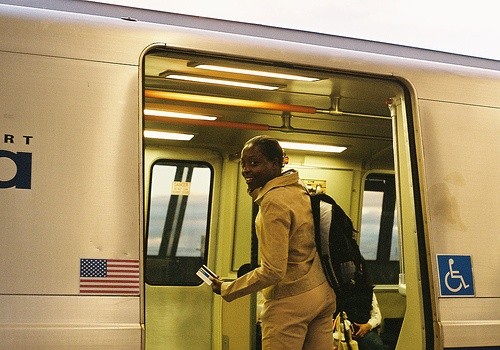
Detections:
[305,188,374,297]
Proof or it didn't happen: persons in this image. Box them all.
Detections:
[345,279,382,350]
[209,135,336,350]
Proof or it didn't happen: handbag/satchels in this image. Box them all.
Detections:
[333,312,359,350]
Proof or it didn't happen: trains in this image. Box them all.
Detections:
[3,0,499,349]
